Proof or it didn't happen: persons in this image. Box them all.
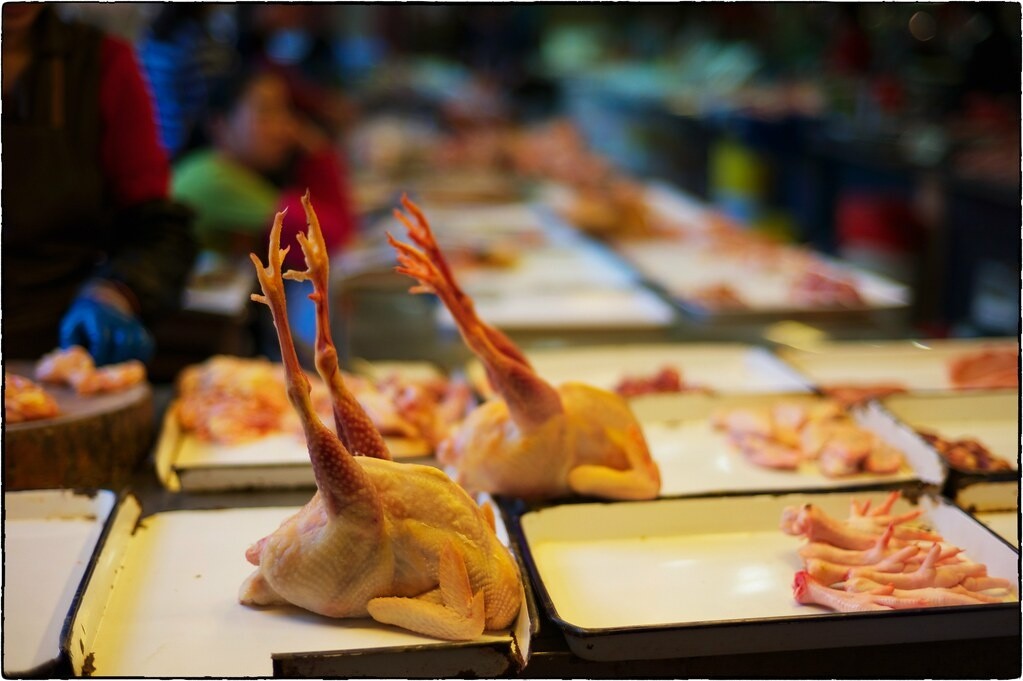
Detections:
[2,1,363,382]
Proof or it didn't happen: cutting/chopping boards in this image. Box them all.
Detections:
[5,360,158,496]
